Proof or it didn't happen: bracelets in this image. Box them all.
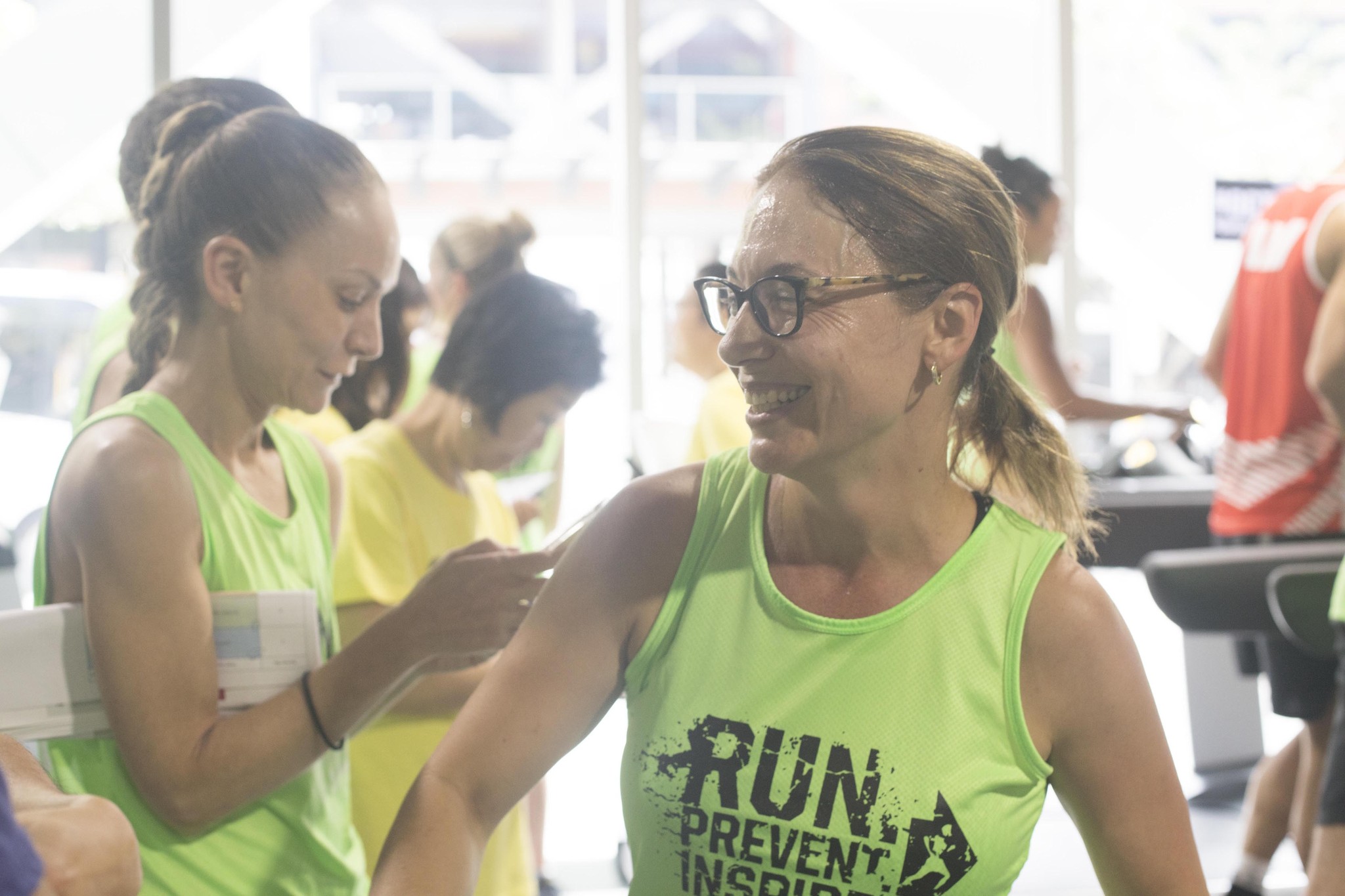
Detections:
[302,674,345,750]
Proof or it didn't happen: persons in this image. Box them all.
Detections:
[669,260,755,465]
[949,145,1198,503]
[0,76,606,896]
[365,128,1207,896]
[1199,165,1345,896]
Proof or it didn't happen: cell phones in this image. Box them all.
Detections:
[540,502,602,561]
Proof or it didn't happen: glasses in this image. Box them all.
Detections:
[693,273,944,338]
